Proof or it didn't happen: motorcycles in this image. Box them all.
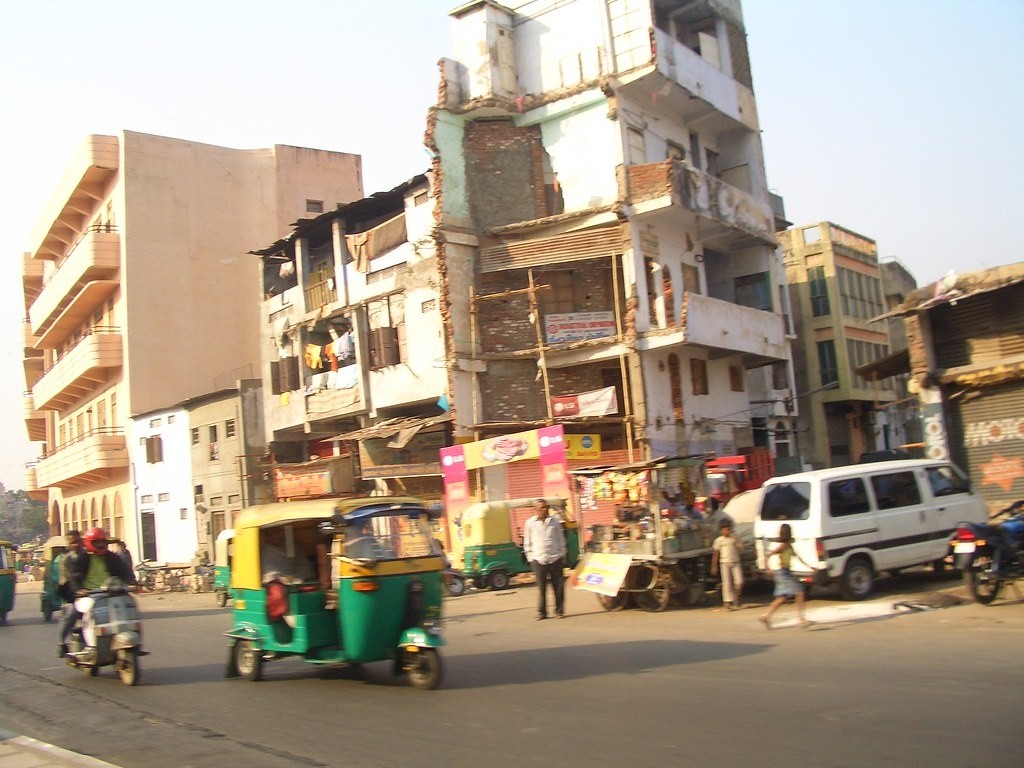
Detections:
[459,497,582,591]
[13,541,47,573]
[222,494,452,690]
[434,538,468,599]
[213,527,234,608]
[948,498,1024,605]
[0,539,21,624]
[38,534,137,622]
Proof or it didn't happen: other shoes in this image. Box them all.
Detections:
[55,644,67,658]
[735,598,741,607]
[800,621,815,628]
[723,602,731,609]
[556,613,564,619]
[759,616,769,628]
[534,612,545,620]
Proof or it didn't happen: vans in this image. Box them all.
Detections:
[753,460,990,602]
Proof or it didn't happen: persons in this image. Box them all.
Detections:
[523,498,567,621]
[711,518,746,612]
[659,489,734,530]
[53,528,137,659]
[759,523,819,631]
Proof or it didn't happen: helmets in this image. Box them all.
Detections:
[84,528,108,555]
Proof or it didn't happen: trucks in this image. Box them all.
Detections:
[703,442,775,510]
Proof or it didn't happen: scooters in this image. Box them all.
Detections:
[59,577,147,687]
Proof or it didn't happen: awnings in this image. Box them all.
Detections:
[960,288,961,289]
[866,273,1024,326]
[853,348,912,382]
[920,354,1024,389]
